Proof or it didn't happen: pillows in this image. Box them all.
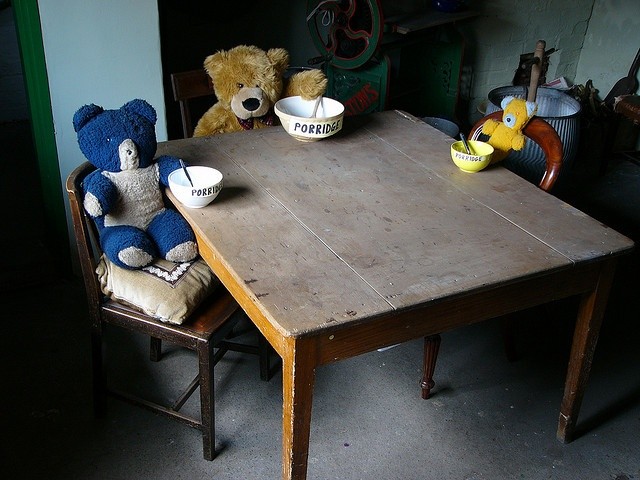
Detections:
[95,252,218,328]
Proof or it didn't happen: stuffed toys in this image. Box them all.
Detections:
[481,95,538,166]
[71,97,195,271]
[191,45,329,137]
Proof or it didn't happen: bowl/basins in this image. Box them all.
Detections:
[168,166,223,208]
[449,139,495,173]
[273,94,346,143]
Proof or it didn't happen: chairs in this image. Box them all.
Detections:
[65,159,272,462]
[418,108,565,400]
[170,68,234,342]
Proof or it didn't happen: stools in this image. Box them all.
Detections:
[614,94,640,177]
